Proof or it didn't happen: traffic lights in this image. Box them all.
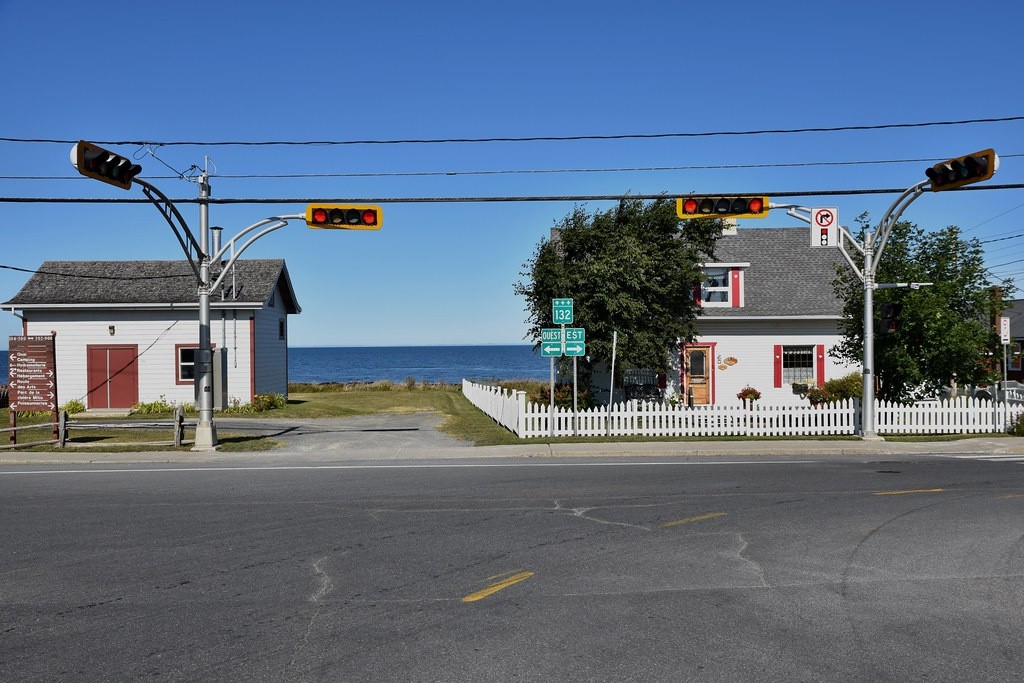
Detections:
[71,140,141,190]
[306,202,382,230]
[925,149,995,194]
[676,196,769,220]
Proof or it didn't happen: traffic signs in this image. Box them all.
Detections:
[9,334,57,412]
[540,329,585,357]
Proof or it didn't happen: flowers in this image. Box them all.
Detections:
[737,384,763,402]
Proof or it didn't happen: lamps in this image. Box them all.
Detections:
[109,326,115,335]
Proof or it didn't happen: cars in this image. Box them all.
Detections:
[972,379,1024,401]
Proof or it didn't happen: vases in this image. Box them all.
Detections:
[743,397,753,412]
